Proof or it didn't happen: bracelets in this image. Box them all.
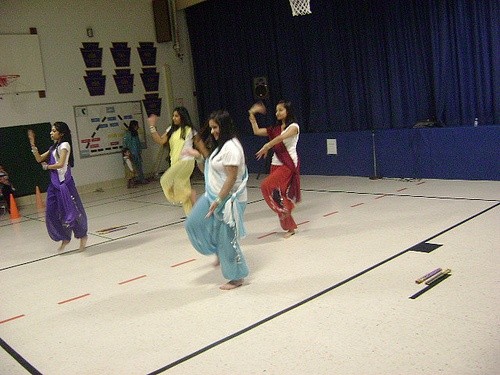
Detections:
[149,126,157,133]
[31,147,37,152]
[33,149,38,155]
[216,196,222,203]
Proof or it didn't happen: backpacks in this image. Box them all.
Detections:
[413,120,434,128]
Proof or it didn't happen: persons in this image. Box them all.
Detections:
[248,101,301,239]
[28,122,88,252]
[175,110,249,290]
[148,107,198,218]
[123,120,150,189]
[0,165,15,214]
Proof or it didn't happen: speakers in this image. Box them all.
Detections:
[252,77,270,100]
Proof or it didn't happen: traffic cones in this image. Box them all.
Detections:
[34,186,46,210]
[9,194,20,218]
